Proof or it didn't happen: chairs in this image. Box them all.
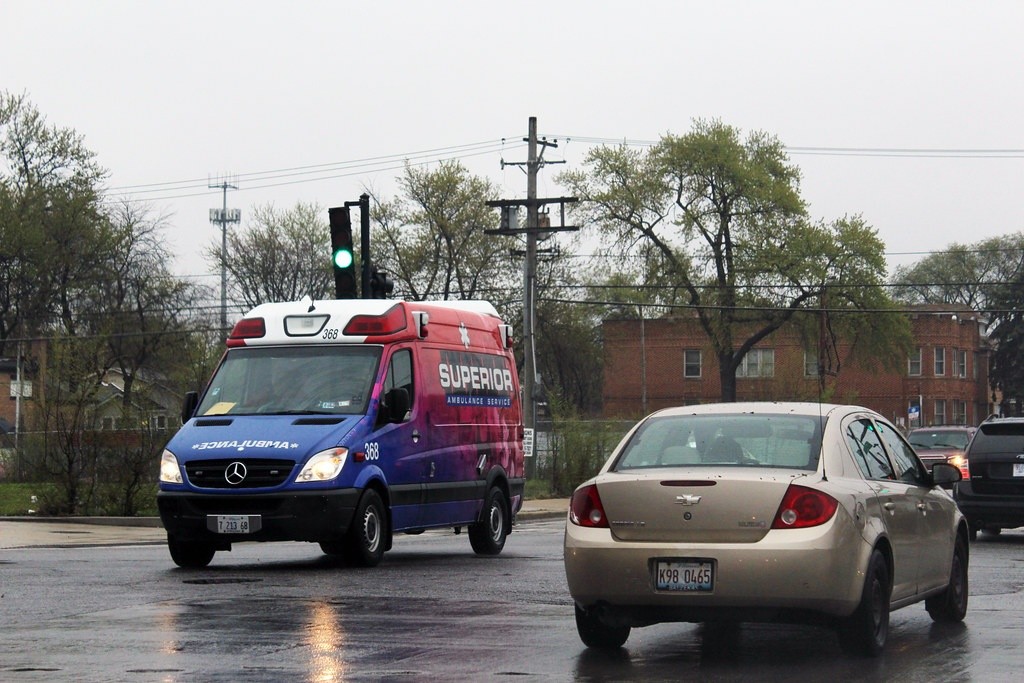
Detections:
[663,437,751,464]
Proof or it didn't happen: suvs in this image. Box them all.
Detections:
[907,424,978,472]
[952,413,1024,539]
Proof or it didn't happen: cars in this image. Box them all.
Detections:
[563,400,970,658]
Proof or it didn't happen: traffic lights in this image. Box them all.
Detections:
[328,207,357,299]
[372,271,394,300]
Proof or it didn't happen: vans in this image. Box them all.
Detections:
[157,299,526,567]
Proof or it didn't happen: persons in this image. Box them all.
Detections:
[257,366,301,411]
[703,437,743,462]
